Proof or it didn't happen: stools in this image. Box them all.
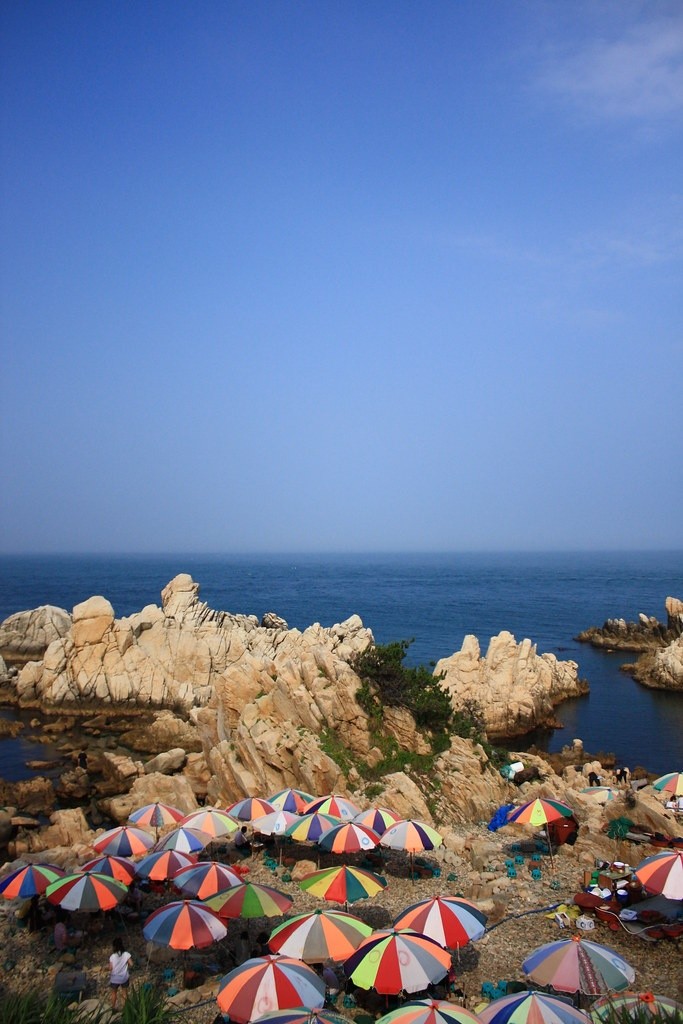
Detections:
[325,994,337,1004]
[3,876,229,998]
[218,836,460,884]
[443,983,456,993]
[481,981,509,1002]
[505,842,549,879]
[343,995,357,1008]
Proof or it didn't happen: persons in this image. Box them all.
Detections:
[108,938,133,1008]
[54,912,67,952]
[313,963,339,985]
[125,880,143,913]
[251,932,279,958]
[234,826,251,851]
[234,930,251,965]
[666,795,683,812]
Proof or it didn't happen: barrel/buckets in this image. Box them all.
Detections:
[545,818,576,844]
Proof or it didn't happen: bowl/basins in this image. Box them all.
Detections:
[652,839,669,847]
[646,923,683,938]
[673,841,683,848]
[597,906,618,923]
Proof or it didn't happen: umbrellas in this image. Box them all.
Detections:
[128,801,188,843]
[379,818,442,886]
[507,796,574,869]
[393,895,488,962]
[588,992,683,1024]
[477,990,593,1024]
[0,788,453,1024]
[299,864,388,914]
[633,848,683,901]
[374,999,480,1024]
[652,770,683,796]
[521,931,634,1008]
